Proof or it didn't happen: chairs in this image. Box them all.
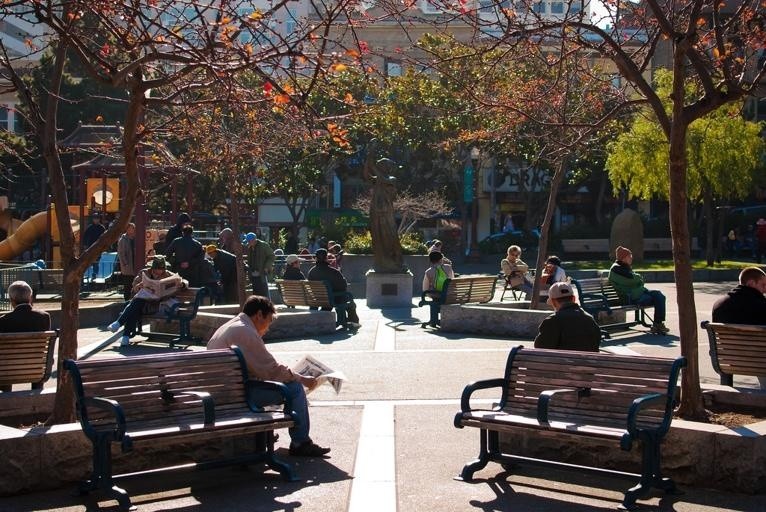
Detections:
[500,270,536,301]
[572,278,666,338]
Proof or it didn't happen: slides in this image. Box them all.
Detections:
[0,210,49,259]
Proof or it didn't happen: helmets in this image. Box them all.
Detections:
[206,245,216,254]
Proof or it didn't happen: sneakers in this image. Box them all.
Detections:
[108,321,120,332]
[121,336,129,345]
[651,324,669,333]
[289,440,330,456]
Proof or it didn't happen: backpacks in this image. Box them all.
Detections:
[431,264,449,291]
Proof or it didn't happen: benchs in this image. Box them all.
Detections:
[0,269,64,300]
[701,321,765,386]
[454,345,687,506]
[277,280,354,322]
[125,287,206,347]
[63,346,299,508]
[419,276,497,328]
[0,329,61,391]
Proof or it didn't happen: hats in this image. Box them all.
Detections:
[616,246,632,260]
[429,252,443,258]
[287,255,305,265]
[246,233,255,241]
[547,282,573,305]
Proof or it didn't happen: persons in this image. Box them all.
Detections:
[422,238,455,326]
[712,267,766,391]
[720,217,766,263]
[539,256,566,302]
[82,211,361,350]
[535,282,602,397]
[608,247,670,332]
[206,296,331,459]
[0,280,51,393]
[501,245,535,308]
